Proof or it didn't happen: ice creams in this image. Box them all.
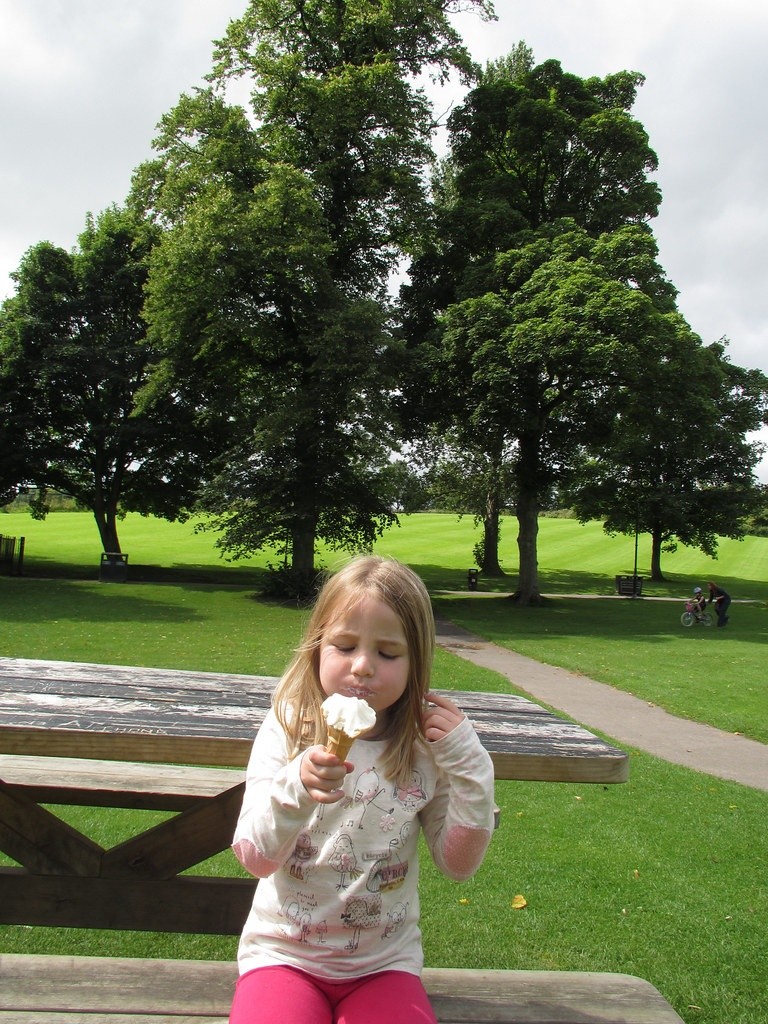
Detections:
[320,693,376,763]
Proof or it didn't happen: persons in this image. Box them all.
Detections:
[687,587,706,621]
[227,556,494,1024]
[706,581,731,628]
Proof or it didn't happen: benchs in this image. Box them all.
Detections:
[0,754,501,831]
[0,951,687,1024]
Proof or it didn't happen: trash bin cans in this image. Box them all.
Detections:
[467,569,478,591]
[98,552,129,583]
[616,575,644,596]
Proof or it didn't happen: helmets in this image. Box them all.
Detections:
[693,587,701,594]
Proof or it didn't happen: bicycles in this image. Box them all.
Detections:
[680,602,714,628]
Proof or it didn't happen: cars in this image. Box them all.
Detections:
[501,490,560,509]
[385,502,404,513]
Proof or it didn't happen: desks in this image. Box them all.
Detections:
[0,652,630,783]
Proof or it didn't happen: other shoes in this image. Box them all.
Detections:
[697,613,702,618]
